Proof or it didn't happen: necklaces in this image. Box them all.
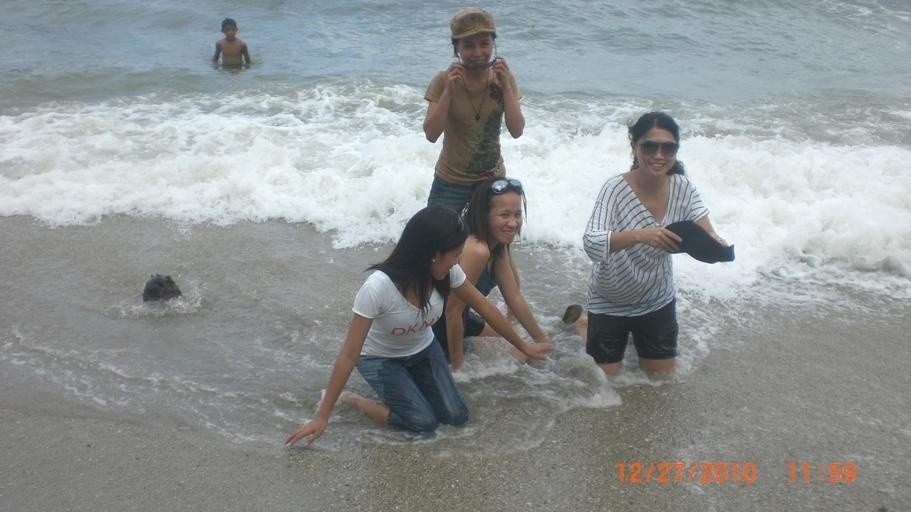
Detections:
[460,67,490,121]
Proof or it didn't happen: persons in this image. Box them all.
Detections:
[423,7,525,215]
[569,111,728,375]
[432,176,555,373]
[212,18,251,63]
[285,205,554,448]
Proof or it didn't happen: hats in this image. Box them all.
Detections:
[450,9,497,41]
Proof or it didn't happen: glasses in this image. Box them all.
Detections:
[490,179,523,194]
[637,140,677,156]
[459,47,498,70]
